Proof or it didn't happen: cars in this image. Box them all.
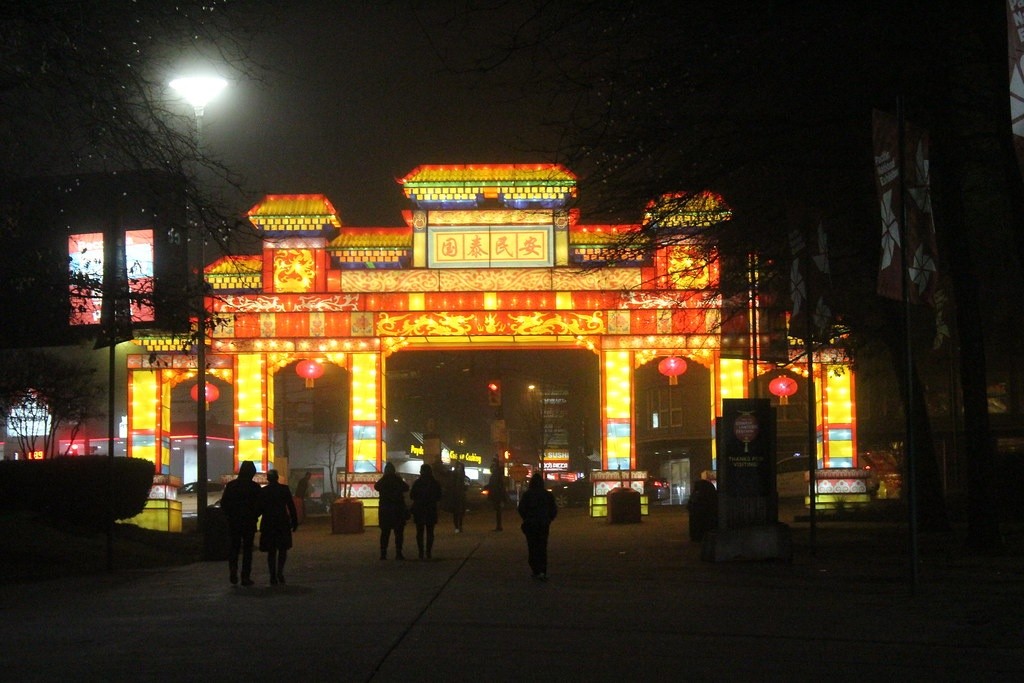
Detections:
[746,450,903,514]
[552,471,677,511]
[175,481,227,517]
[464,475,490,505]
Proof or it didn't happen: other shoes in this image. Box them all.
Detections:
[395,555,404,560]
[270,572,277,584]
[242,578,254,585]
[278,571,284,579]
[230,566,238,584]
[380,556,385,560]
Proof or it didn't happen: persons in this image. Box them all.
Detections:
[481,457,503,532]
[296,471,311,499]
[374,462,410,560]
[259,468,298,585]
[411,463,441,558]
[443,462,465,533]
[517,473,558,579]
[220,460,263,586]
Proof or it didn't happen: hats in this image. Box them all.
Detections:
[385,462,395,473]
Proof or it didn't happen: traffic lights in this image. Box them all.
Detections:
[487,379,501,406]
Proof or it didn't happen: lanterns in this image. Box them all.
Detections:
[296,359,324,388]
[769,374,798,405]
[191,381,219,411]
[658,356,687,385]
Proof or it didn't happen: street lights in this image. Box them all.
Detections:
[166,72,228,539]
[528,384,545,480]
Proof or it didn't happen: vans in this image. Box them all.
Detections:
[396,460,426,512]
